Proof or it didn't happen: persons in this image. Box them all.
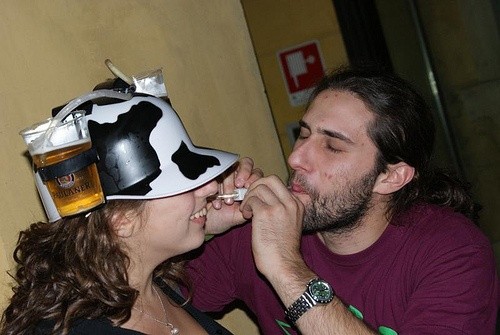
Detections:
[0,59,240,335]
[160,62,500,335]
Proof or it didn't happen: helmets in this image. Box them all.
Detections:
[66,84,240,199]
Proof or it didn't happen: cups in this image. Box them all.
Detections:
[19,110,106,218]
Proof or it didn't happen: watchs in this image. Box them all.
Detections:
[285,276,335,324]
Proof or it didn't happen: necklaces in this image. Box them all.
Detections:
[132,283,181,334]
